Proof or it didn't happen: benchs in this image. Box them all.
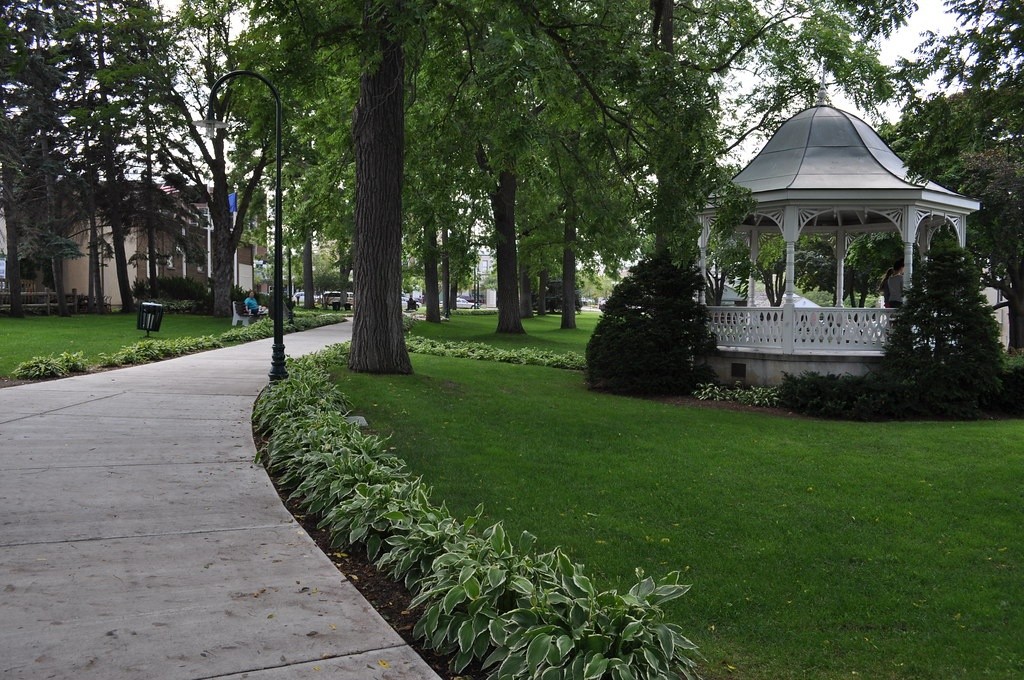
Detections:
[232,301,257,326]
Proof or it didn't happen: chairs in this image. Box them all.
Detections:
[104,295,113,313]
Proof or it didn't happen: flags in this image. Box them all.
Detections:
[227,192,237,212]
[159,180,180,194]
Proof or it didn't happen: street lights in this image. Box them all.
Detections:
[189,69,290,387]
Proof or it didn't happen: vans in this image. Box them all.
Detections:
[323,292,354,306]
[292,292,319,303]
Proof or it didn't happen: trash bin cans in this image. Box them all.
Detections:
[332,302,340,311]
[137,301,164,332]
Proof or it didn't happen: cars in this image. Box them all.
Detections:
[401,297,422,311]
[440,298,478,309]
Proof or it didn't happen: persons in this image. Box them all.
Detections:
[408,298,416,309]
[879,259,904,307]
[245,291,260,314]
